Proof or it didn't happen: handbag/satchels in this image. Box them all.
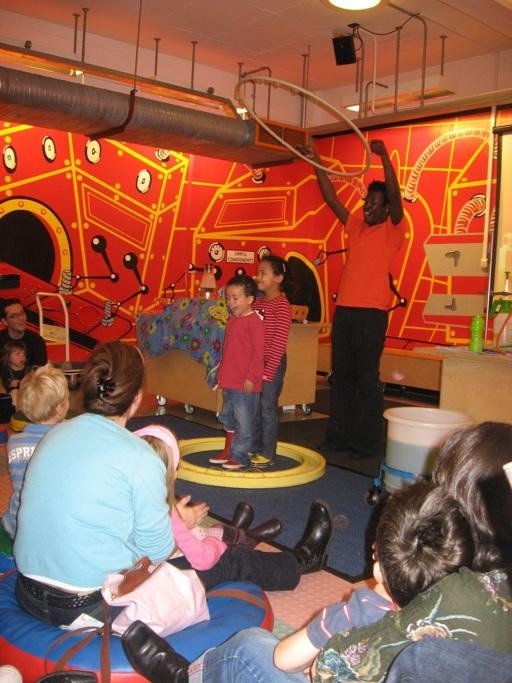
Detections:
[101,557,210,638]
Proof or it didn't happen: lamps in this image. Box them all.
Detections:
[332,24,359,65]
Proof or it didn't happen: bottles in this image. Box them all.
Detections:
[470,314,483,354]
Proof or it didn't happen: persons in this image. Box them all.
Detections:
[120,420,512,682]
[297,139,406,458]
[129,424,282,572]
[1,362,70,542]
[248,255,293,466]
[208,275,265,469]
[2,339,32,393]
[13,341,334,631]
[273,480,474,676]
[0,297,48,368]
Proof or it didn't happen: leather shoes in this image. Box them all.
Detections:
[121,619,192,683]
[36,672,98,683]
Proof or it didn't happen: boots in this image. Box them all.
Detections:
[208,431,249,468]
[212,501,283,551]
[293,498,334,574]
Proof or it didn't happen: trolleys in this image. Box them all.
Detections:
[35,291,84,389]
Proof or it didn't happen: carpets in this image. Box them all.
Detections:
[125,389,418,583]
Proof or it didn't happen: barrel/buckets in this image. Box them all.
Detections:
[382,405,472,494]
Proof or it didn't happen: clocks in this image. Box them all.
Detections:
[3,144,16,172]
[207,242,225,263]
[136,168,151,193]
[42,136,56,162]
[85,139,101,163]
[257,245,271,261]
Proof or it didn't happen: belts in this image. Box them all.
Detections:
[17,572,103,609]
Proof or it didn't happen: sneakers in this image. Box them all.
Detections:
[1,664,24,682]
[249,452,274,468]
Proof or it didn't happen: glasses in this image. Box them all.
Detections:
[7,310,25,319]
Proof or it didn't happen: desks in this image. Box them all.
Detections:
[317,343,512,426]
[142,317,319,422]
